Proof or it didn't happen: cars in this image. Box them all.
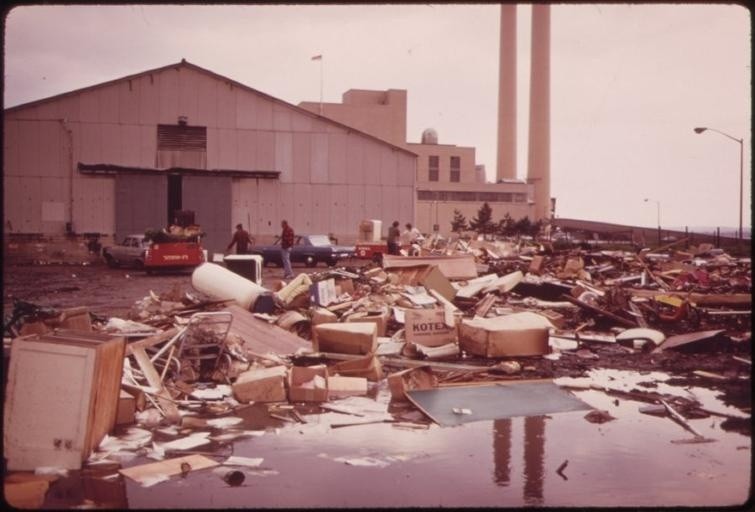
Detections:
[248,233,358,268]
[103,235,154,271]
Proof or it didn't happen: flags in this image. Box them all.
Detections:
[312,55,321,60]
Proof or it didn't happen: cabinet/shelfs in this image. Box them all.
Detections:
[5,326,124,472]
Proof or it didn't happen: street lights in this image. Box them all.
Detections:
[694,127,743,257]
[645,198,662,248]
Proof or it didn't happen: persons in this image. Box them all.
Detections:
[388,221,401,255]
[224,224,252,255]
[280,220,294,278]
[403,223,424,255]
